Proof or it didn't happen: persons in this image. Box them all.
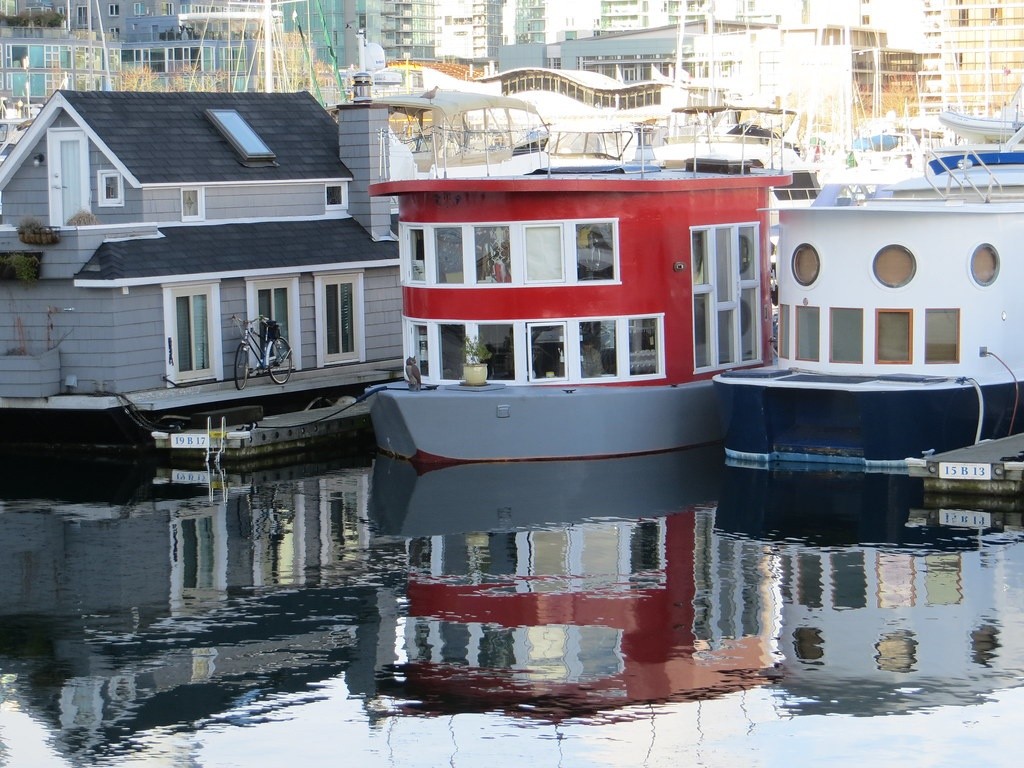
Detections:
[579,334,604,377]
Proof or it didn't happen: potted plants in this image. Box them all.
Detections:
[459,332,492,386]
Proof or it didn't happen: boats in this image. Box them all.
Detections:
[361,107,823,470]
[364,49,1024,357]
[713,101,1024,468]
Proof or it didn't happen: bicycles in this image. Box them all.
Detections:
[231,312,295,391]
